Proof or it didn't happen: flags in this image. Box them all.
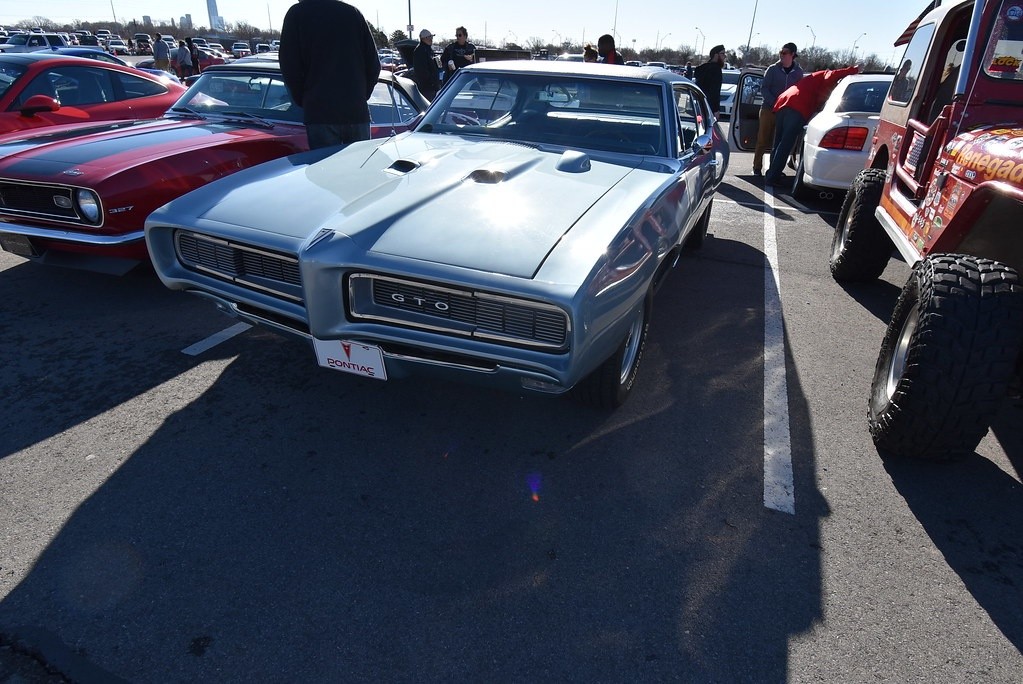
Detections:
[893,0,941,47]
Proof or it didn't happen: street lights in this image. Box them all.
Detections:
[552,30,561,44]
[660,33,671,51]
[695,27,706,54]
[806,25,816,46]
[611,28,621,47]
[851,32,867,54]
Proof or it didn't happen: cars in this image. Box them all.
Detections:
[0,51,231,137]
[728,69,897,200]
[1,21,743,141]
[0,62,435,270]
[142,60,729,406]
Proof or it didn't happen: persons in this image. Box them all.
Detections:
[598,35,624,65]
[177,37,201,79]
[685,62,692,80]
[440,26,480,90]
[765,63,864,187]
[153,33,171,71]
[278,0,381,148]
[584,45,598,62]
[414,29,442,101]
[753,43,803,175]
[138,42,146,55]
[890,59,912,101]
[694,44,726,119]
[128,38,134,55]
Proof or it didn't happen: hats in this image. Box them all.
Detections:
[710,45,724,57]
[784,43,798,58]
[419,30,435,38]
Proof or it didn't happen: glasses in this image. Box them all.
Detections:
[455,33,463,37]
[779,51,791,55]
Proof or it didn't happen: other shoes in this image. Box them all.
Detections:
[765,180,784,188]
[752,168,761,176]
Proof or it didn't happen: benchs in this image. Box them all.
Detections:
[367,104,413,123]
[506,113,697,155]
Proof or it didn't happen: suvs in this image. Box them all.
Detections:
[827,1,1023,471]
